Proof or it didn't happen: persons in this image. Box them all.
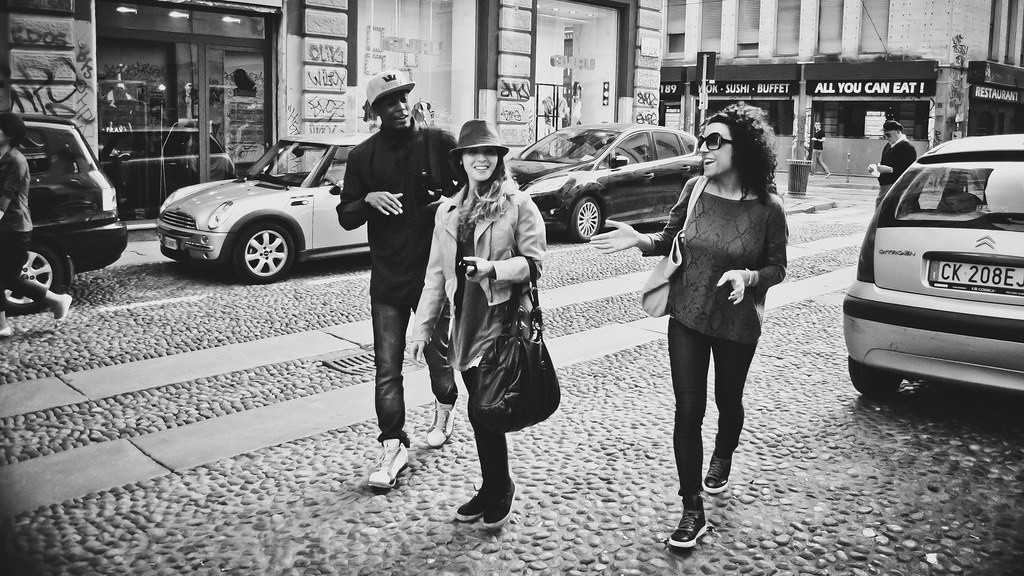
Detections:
[409,120,548,528]
[336,71,467,488]
[810,122,831,179]
[868,120,917,212]
[589,102,788,548]
[0,113,73,338]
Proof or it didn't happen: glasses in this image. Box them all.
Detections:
[699,132,732,150]
[883,133,892,139]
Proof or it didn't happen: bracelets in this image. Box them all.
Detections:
[817,139,818,141]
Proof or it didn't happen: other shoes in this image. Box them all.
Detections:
[54,294,73,327]
[0,326,11,336]
[825,173,831,178]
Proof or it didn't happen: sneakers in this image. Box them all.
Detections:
[669,498,707,548]
[427,395,458,448]
[368,439,409,489]
[703,449,732,493]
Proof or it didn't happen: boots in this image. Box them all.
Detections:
[455,434,517,528]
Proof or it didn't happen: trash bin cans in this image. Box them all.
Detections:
[786,159,811,194]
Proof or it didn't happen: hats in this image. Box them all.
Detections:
[365,69,415,106]
[883,121,903,132]
[449,119,509,155]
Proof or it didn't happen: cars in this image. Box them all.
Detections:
[156,132,375,286]
[4,113,129,317]
[843,134,1024,398]
[506,122,704,243]
[98,126,235,210]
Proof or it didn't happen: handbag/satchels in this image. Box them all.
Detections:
[474,256,561,434]
[641,175,709,317]
